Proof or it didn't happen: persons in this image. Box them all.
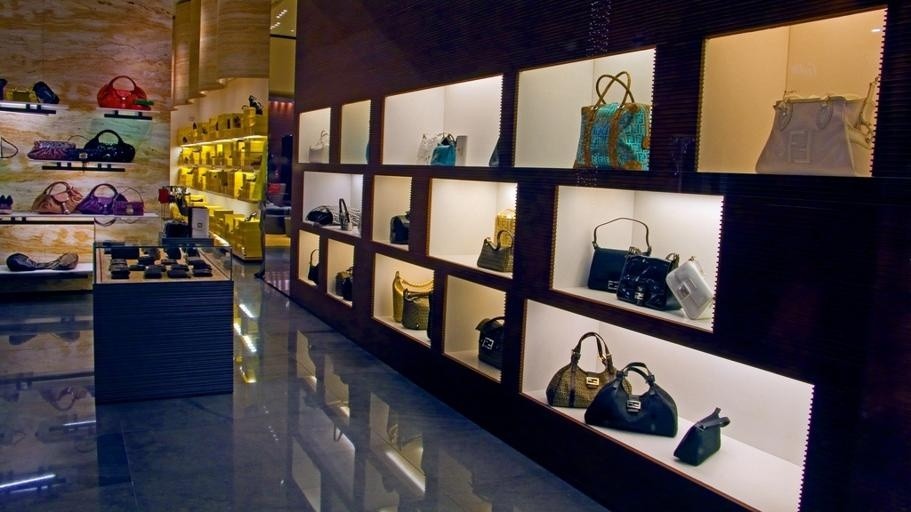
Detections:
[253,184,266,279]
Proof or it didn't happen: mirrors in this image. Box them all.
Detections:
[263,0,298,298]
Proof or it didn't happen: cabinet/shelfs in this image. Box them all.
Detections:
[288,1,886,510]
[0,101,159,291]
[178,136,266,261]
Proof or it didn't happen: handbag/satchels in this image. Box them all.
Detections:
[755,76,878,177]
[308,249,320,285]
[546,332,729,466]
[96,76,146,109]
[33,81,59,104]
[6,251,78,271]
[572,72,651,171]
[309,128,330,164]
[416,131,456,166]
[336,267,354,301]
[393,270,434,340]
[249,96,265,114]
[587,217,716,320]
[390,210,411,243]
[475,317,507,371]
[28,129,143,215]
[305,198,360,230]
[477,208,516,272]
